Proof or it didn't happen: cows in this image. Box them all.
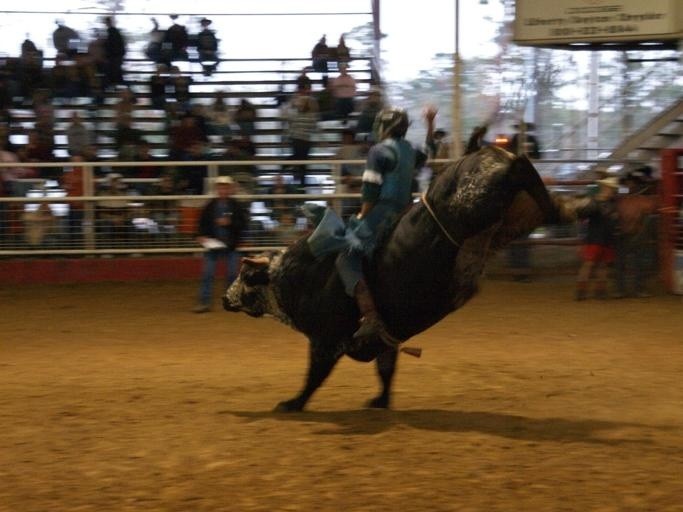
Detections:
[221,105,617,414]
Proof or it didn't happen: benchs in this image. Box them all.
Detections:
[0,56,382,260]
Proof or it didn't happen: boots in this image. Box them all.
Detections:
[353,279,379,338]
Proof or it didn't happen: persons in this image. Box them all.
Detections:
[334,105,418,340]
[506,115,541,160]
[421,105,450,193]
[0,13,257,250]
[462,116,492,153]
[190,174,245,313]
[266,36,383,226]
[568,164,659,302]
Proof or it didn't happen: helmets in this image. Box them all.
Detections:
[377,110,409,138]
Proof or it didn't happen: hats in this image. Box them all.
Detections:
[213,175,235,186]
